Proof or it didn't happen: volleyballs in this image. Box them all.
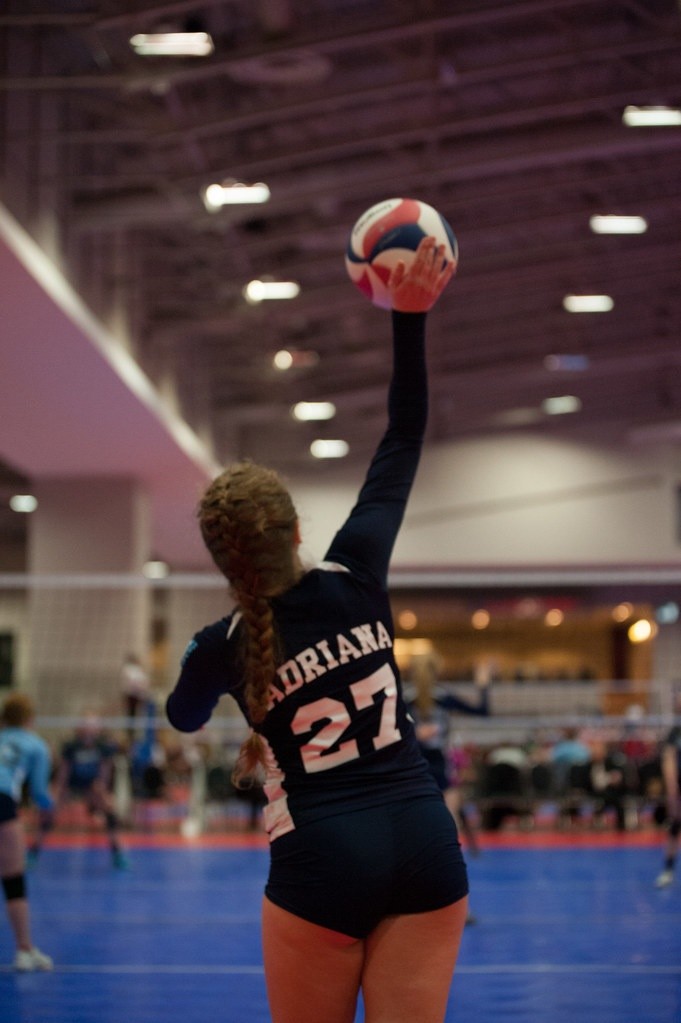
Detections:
[345,194,460,310]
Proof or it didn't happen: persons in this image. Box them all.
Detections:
[165,234,469,1023]
[1,691,54,974]
[125,694,162,799]
[31,702,125,870]
[411,706,462,849]
[477,723,665,837]
[656,723,681,889]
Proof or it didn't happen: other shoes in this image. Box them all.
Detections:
[13,949,54,973]
